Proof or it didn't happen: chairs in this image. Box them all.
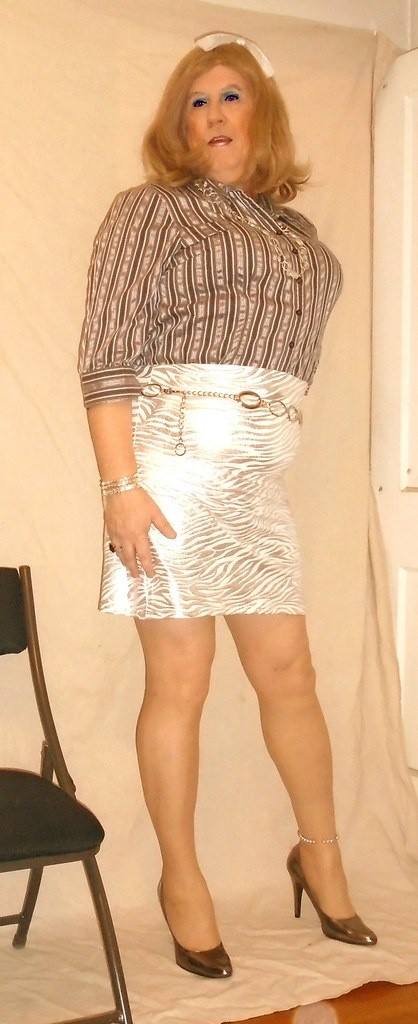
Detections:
[0,566,138,1024]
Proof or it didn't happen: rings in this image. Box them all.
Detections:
[109,543,123,553]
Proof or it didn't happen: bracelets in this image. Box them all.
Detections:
[100,472,140,495]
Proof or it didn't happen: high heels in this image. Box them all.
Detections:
[157,874,233,979]
[286,842,378,946]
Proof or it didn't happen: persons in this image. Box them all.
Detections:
[77,31,378,980]
